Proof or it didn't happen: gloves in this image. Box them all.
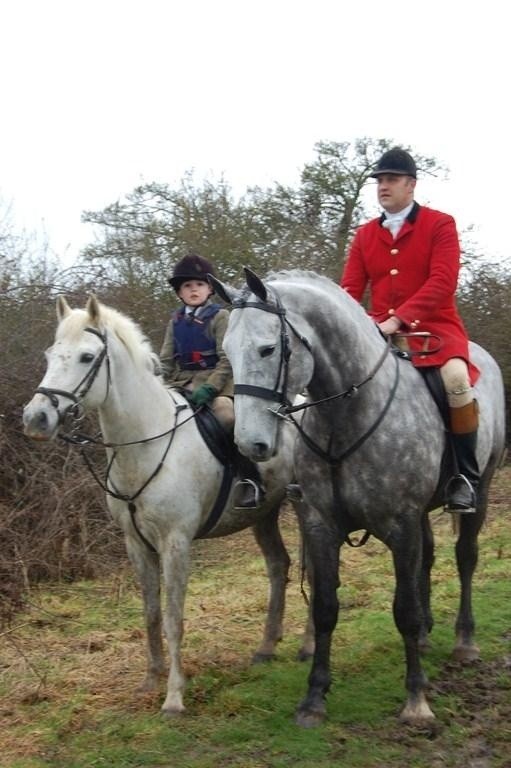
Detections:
[191,383,216,405]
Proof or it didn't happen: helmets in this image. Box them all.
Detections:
[368,150,416,177]
[169,253,214,283]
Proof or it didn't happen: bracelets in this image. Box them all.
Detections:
[390,316,401,328]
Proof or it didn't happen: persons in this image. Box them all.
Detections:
[340,144,481,513]
[156,254,270,509]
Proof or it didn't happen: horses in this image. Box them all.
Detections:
[22,293,315,721]
[207,267,505,739]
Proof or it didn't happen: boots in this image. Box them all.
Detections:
[445,427,480,509]
[243,469,266,506]
